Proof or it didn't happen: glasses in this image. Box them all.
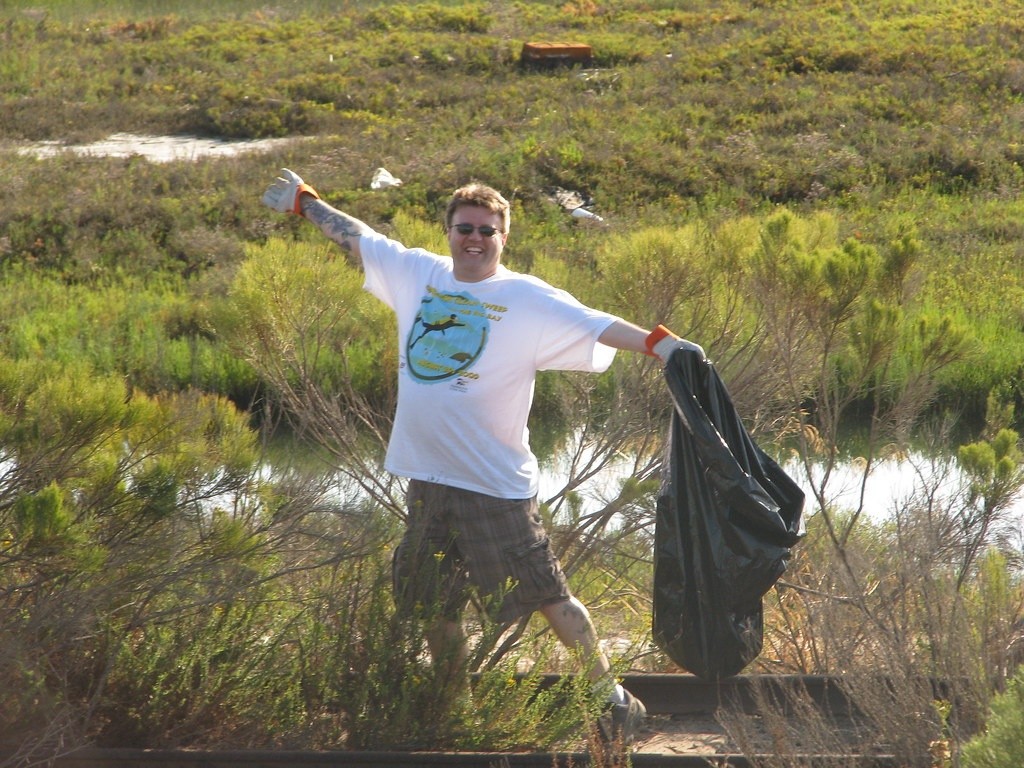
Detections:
[451,223,502,237]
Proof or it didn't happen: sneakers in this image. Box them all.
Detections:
[597,689,647,745]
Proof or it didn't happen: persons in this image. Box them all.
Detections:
[258,166,714,755]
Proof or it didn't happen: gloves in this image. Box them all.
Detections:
[263,168,320,217]
[644,325,706,364]
